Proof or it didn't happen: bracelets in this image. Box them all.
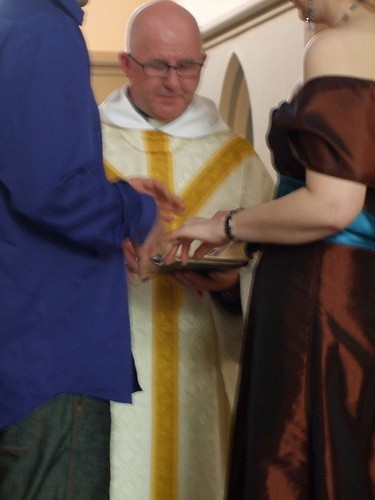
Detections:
[224,207,248,244]
[208,278,244,300]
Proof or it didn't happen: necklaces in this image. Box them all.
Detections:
[332,1,363,28]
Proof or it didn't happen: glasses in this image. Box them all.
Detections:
[125,52,206,79]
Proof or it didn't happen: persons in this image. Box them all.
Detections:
[94,0,273,499]
[159,0,375,500]
[0,0,185,500]
